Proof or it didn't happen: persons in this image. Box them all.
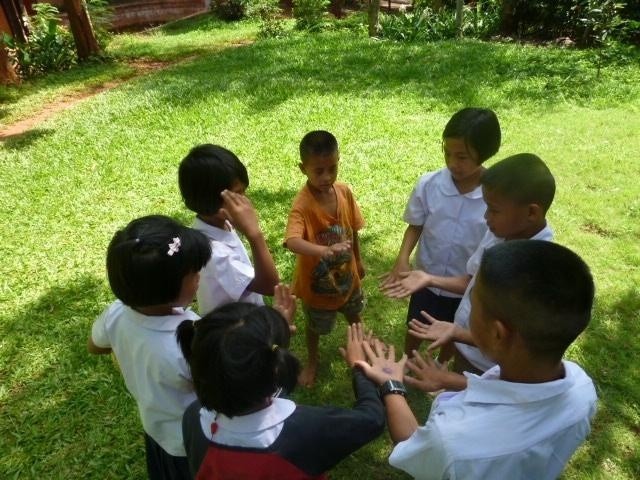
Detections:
[88,216,296,478]
[354,240,598,479]
[178,145,279,318]
[383,152,560,378]
[283,132,367,390]
[175,303,386,479]
[378,107,503,373]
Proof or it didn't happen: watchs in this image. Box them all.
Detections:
[377,381,408,396]
